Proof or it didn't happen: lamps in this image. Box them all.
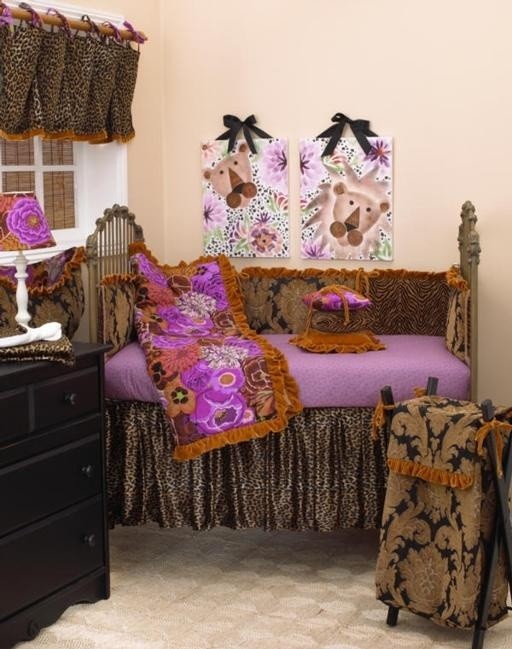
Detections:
[0,191,56,330]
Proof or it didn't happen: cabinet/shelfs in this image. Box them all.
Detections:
[0,342,114,648]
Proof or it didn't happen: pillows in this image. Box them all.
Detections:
[309,308,376,331]
[303,286,375,311]
[287,330,389,354]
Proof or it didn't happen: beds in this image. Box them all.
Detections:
[85,200,482,537]
